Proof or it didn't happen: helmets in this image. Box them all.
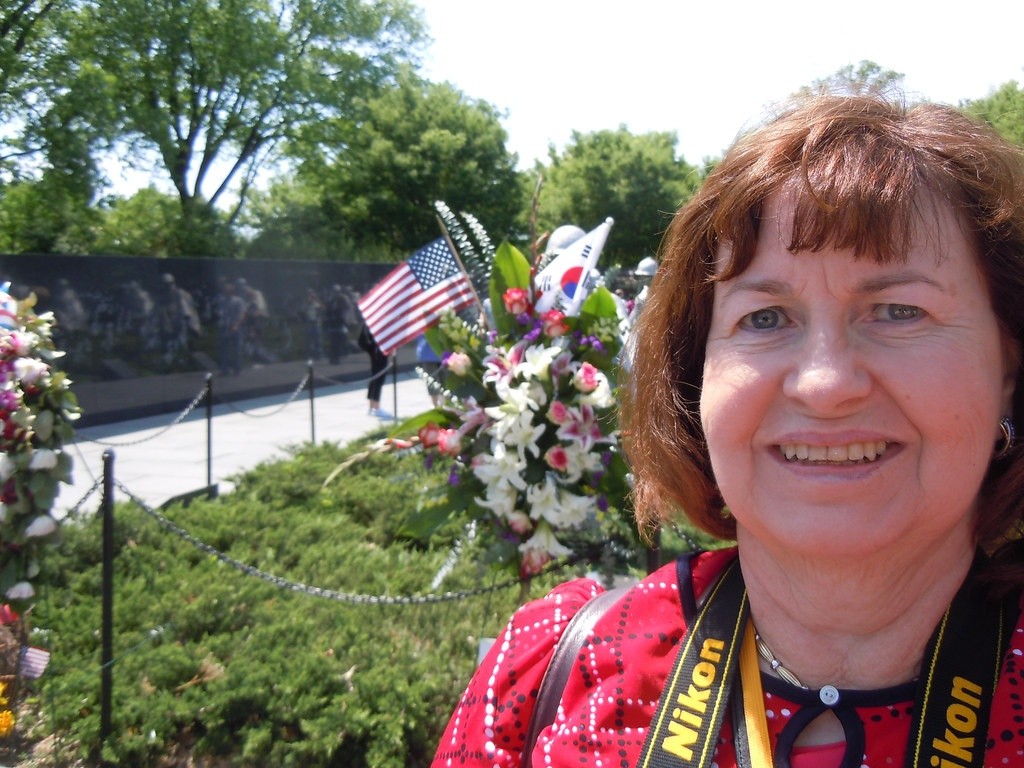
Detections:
[634,257,656,276]
[546,225,586,257]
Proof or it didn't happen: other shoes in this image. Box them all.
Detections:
[369,407,389,417]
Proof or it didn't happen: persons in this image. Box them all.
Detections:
[421,94,1023,768]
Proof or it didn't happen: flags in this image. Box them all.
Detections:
[530,223,605,327]
[355,238,476,358]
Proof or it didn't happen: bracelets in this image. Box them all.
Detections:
[754,630,809,691]
